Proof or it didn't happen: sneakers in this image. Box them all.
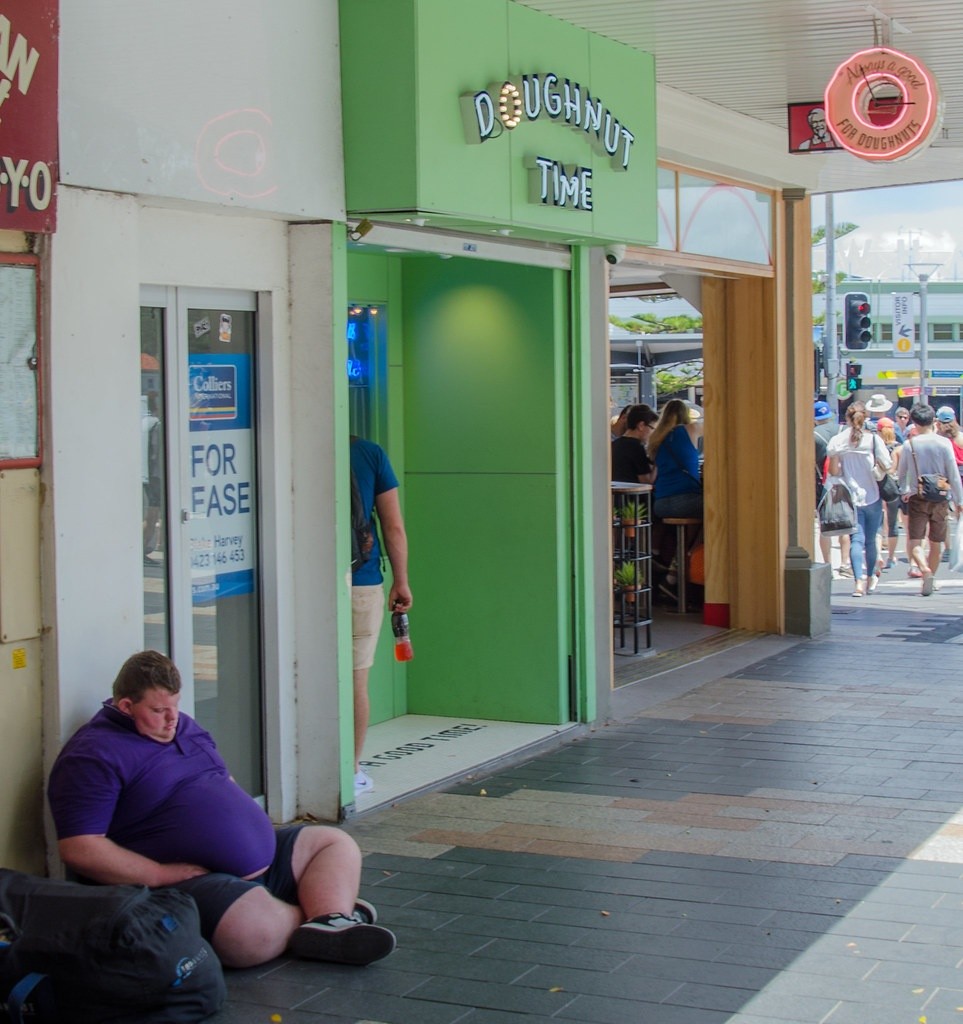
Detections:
[353,768,374,796]
[351,896,379,923]
[292,913,398,969]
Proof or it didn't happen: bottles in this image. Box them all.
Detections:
[391,600,413,661]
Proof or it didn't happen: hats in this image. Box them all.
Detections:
[813,401,833,421]
[864,393,894,413]
[877,417,894,431]
[936,406,956,423]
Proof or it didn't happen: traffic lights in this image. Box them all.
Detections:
[844,293,871,350]
[846,363,862,392]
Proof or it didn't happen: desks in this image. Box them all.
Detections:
[611,481,653,655]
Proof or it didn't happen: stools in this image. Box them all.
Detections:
[661,516,703,617]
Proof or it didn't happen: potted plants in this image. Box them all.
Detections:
[614,560,645,602]
[612,507,618,520]
[621,501,648,537]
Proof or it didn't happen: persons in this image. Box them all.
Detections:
[814,394,963,597]
[611,400,704,603]
[351,433,412,799]
[47,649,395,970]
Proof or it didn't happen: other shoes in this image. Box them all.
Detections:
[875,556,885,575]
[908,565,923,577]
[886,557,898,568]
[931,581,938,591]
[867,574,878,594]
[658,578,681,600]
[839,566,854,578]
[650,552,669,569]
[852,588,865,596]
[941,548,952,562]
[882,537,889,549]
[921,572,934,596]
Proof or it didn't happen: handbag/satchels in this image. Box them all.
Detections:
[817,476,857,537]
[917,473,953,503]
[948,511,963,574]
[877,473,901,503]
[0,866,228,1024]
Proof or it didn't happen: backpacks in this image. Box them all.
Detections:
[350,470,375,569]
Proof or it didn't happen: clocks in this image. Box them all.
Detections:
[824,48,938,161]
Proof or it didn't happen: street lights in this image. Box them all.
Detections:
[908,264,941,405]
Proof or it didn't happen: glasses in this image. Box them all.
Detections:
[898,414,907,420]
